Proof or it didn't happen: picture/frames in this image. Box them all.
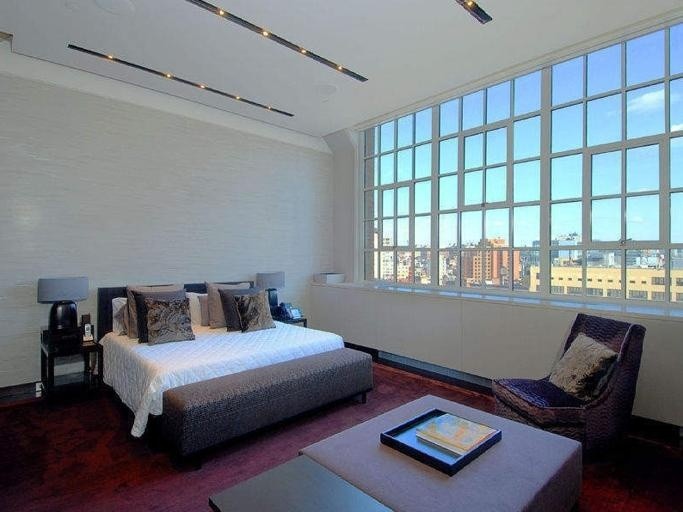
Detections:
[112,281,276,345]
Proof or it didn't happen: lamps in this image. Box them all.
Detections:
[37,278,88,335]
[258,272,284,304]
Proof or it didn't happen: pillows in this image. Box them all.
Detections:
[547,330,618,402]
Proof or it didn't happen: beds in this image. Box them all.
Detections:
[95,281,372,466]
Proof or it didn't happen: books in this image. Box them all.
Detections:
[416,413,497,456]
[281,302,301,320]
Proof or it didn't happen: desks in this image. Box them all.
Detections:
[298,393,582,511]
[208,454,391,512]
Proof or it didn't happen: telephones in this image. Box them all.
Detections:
[82,324,94,342]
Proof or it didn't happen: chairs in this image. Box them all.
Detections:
[490,314,645,463]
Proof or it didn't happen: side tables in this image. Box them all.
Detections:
[39,343,103,404]
[278,316,307,329]
